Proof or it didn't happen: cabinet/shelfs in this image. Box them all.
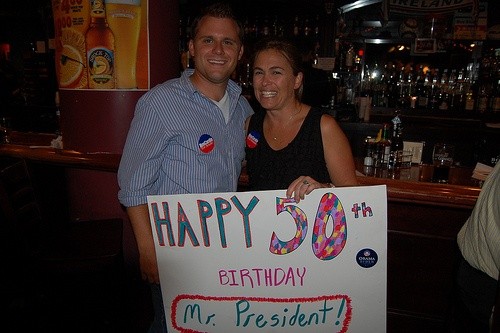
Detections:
[331,0,500,176]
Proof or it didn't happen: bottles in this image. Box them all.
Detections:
[84,0,116,89]
[55,92,61,136]
[167,0,322,71]
[387,123,403,179]
[379,123,392,170]
[235,60,254,95]
[326,48,500,124]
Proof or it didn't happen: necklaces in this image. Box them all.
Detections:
[272,135,278,141]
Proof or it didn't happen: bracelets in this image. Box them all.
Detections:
[327,182,335,188]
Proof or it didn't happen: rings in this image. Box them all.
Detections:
[303,181,310,186]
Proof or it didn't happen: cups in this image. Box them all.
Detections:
[432,143,454,183]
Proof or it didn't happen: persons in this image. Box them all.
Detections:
[117,4,255,333]
[245,38,359,203]
[456,159,500,333]
